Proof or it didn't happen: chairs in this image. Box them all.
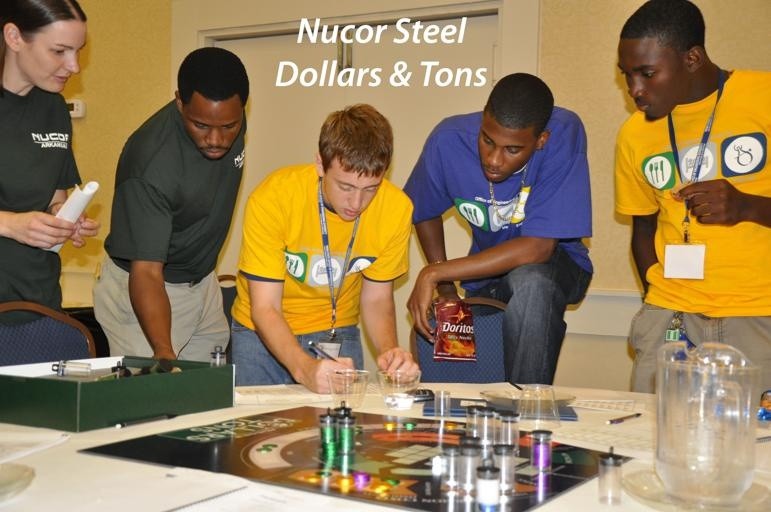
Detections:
[1,299,98,365]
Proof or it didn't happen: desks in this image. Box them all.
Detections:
[2,381,769,507]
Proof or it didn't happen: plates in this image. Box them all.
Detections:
[620,468,771,512]
[478,388,577,410]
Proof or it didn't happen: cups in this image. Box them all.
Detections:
[656,340,758,508]
[376,370,422,411]
[515,382,562,430]
[433,390,451,418]
[326,368,371,408]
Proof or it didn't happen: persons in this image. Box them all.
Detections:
[229,104,422,386]
[404,72,593,385]
[0,1,99,366]
[614,0,771,405]
[92,47,250,363]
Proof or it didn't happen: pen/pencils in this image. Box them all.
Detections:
[508,381,522,392]
[607,413,642,425]
[308,340,338,362]
[756,436,771,441]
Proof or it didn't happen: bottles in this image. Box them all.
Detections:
[50,358,92,378]
[319,400,356,455]
[208,344,227,368]
[429,405,553,512]
[598,450,624,509]
